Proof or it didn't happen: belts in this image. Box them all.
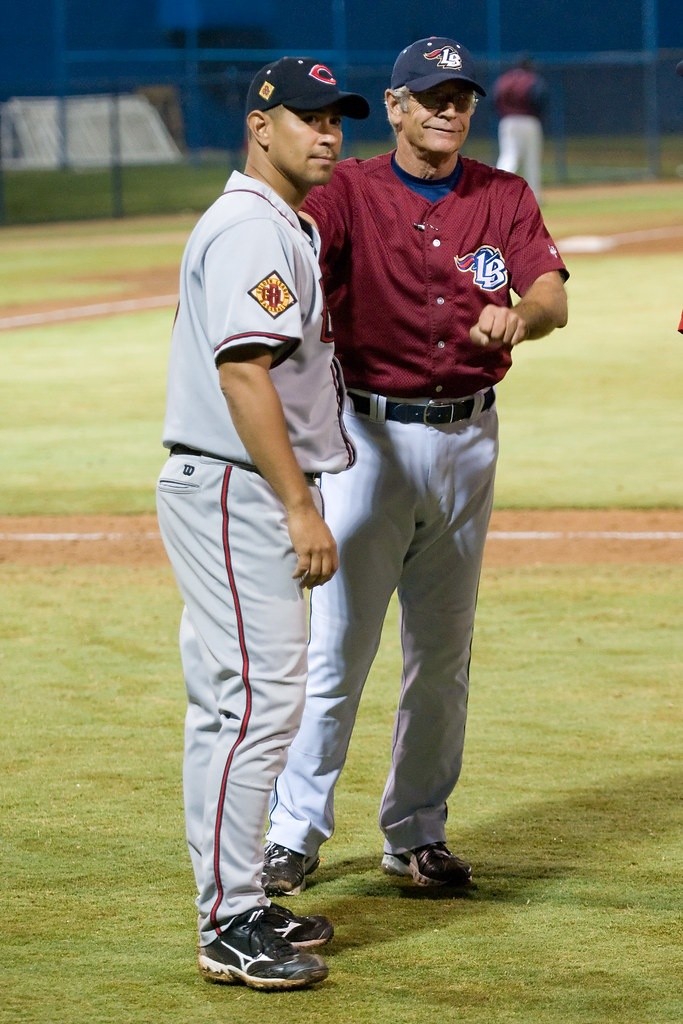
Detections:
[171,444,322,482]
[348,387,497,425]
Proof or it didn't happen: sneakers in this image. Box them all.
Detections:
[199,905,329,990]
[381,842,473,886]
[263,903,333,949]
[260,841,322,899]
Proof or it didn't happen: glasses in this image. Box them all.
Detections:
[417,89,480,114]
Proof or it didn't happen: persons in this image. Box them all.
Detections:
[153,56,371,987]
[252,37,570,902]
[492,51,548,197]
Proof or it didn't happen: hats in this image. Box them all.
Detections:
[248,56,371,120]
[390,35,488,98]
